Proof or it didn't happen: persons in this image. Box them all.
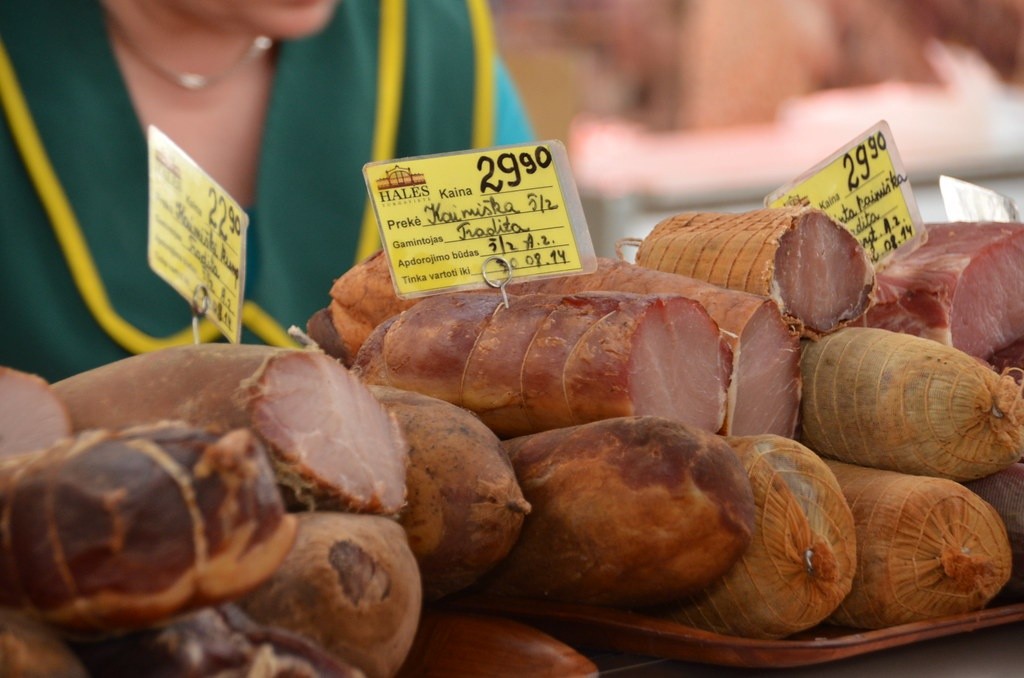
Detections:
[0,0,539,390]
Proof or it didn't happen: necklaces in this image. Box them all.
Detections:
[106,14,274,91]
